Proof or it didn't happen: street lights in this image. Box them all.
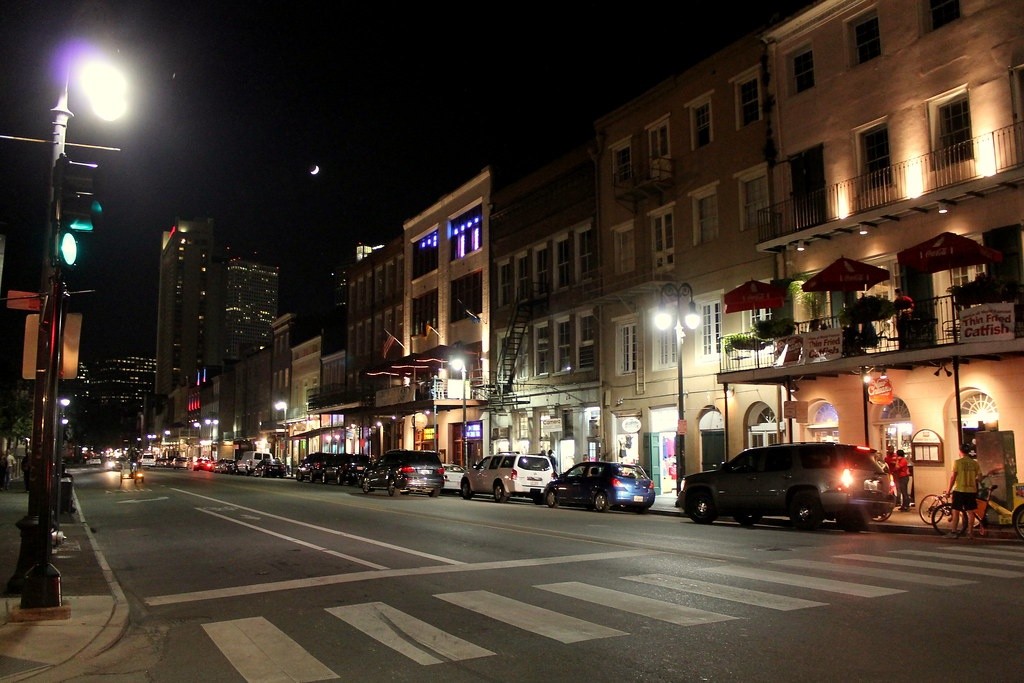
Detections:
[276,402,287,469]
[450,359,468,472]
[193,420,219,460]
[654,283,701,507]
[137,430,170,458]
[3,41,135,596]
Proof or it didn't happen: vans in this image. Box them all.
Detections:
[235,452,272,476]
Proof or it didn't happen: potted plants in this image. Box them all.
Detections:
[754,316,798,339]
[837,293,898,346]
[946,272,1019,308]
[730,331,766,351]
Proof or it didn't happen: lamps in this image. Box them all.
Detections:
[788,382,799,392]
[727,388,735,397]
[797,240,805,252]
[880,368,887,380]
[859,223,869,234]
[933,362,953,378]
[937,201,949,214]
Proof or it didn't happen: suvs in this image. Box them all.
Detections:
[138,454,156,467]
[461,452,558,504]
[297,449,443,497]
[677,441,897,532]
[253,458,287,477]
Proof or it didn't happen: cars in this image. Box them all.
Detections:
[543,461,655,512]
[85,455,129,470]
[214,458,234,473]
[442,464,467,490]
[156,457,214,472]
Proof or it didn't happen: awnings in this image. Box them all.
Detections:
[283,426,345,477]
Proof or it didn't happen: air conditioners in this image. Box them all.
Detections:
[493,426,508,438]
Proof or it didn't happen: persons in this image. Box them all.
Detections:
[547,450,558,473]
[578,454,592,477]
[891,450,911,510]
[941,444,980,543]
[127,444,143,477]
[885,445,901,506]
[0,449,16,490]
[21,446,31,493]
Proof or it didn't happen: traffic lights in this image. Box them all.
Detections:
[52,152,101,268]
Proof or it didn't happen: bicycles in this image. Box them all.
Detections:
[918,485,1024,541]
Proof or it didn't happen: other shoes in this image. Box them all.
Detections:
[898,506,910,511]
[942,532,957,538]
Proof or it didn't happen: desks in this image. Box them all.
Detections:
[897,317,938,351]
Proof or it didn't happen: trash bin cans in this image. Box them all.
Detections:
[60,473,73,514]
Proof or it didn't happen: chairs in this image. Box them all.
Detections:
[591,468,599,476]
